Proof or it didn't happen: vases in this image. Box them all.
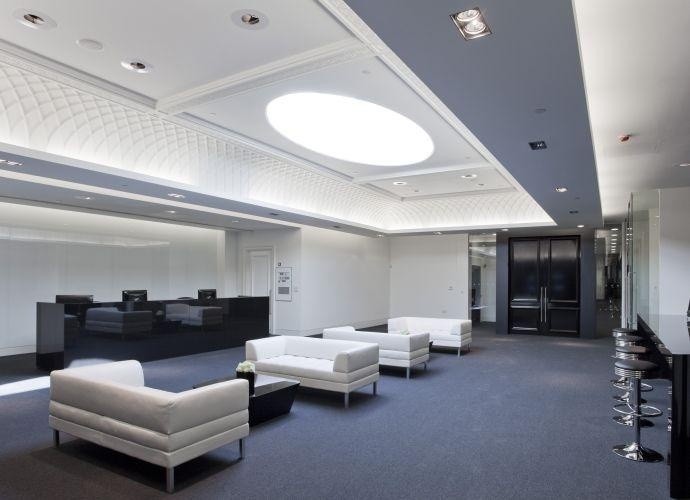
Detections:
[236,371,256,396]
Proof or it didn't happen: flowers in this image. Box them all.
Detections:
[235,360,257,374]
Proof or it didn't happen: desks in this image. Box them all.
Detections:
[634,310,690,500]
[193,370,301,428]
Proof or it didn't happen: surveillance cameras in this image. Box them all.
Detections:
[619,135,629,141]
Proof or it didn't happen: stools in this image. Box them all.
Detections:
[606,318,666,469]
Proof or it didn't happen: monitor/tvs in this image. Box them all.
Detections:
[56,295,93,303]
[122,290,147,302]
[198,289,216,299]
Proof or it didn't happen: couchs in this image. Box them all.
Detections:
[239,331,381,410]
[44,358,250,494]
[384,315,475,356]
[320,323,431,378]
[52,295,225,335]
